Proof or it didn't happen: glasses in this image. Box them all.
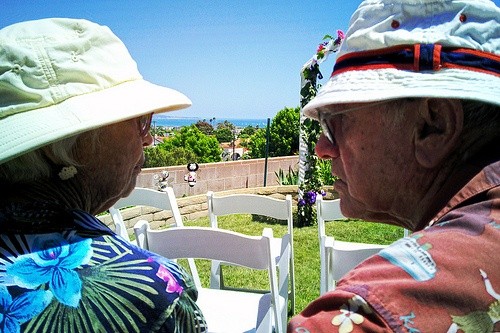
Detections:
[137,112,156,137]
[318,98,409,147]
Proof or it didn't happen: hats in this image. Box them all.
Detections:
[301,1,500,121]
[0,17,192,167]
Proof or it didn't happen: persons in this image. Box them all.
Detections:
[0,18,209,333]
[285,0,500,333]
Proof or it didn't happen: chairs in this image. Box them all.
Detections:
[108,187,408,333]
